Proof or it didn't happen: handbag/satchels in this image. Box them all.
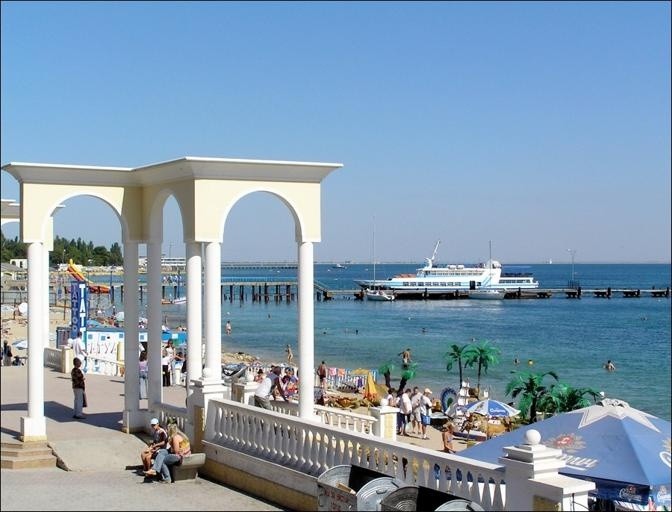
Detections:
[83,391,87,407]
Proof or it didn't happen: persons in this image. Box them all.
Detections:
[443,421,456,453]
[2,339,11,367]
[84,302,186,333]
[225,320,231,336]
[509,419,514,427]
[12,355,25,366]
[138,418,167,477]
[138,338,190,401]
[162,272,183,283]
[142,418,195,484]
[284,343,293,364]
[602,358,616,371]
[459,414,474,432]
[503,420,510,432]
[70,357,87,420]
[251,358,442,441]
[398,348,413,367]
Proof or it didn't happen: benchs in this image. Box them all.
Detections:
[147,436,206,482]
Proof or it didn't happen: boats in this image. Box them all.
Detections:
[353,240,539,301]
[171,295,186,305]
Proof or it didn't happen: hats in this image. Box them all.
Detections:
[424,388,433,394]
[149,417,160,426]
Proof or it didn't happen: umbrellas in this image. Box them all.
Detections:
[422,396,672,512]
[454,397,522,441]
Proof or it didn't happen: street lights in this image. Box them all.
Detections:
[63,249,66,277]
[567,248,576,281]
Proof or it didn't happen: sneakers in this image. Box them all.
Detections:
[142,468,157,475]
[159,478,171,484]
[72,414,86,419]
